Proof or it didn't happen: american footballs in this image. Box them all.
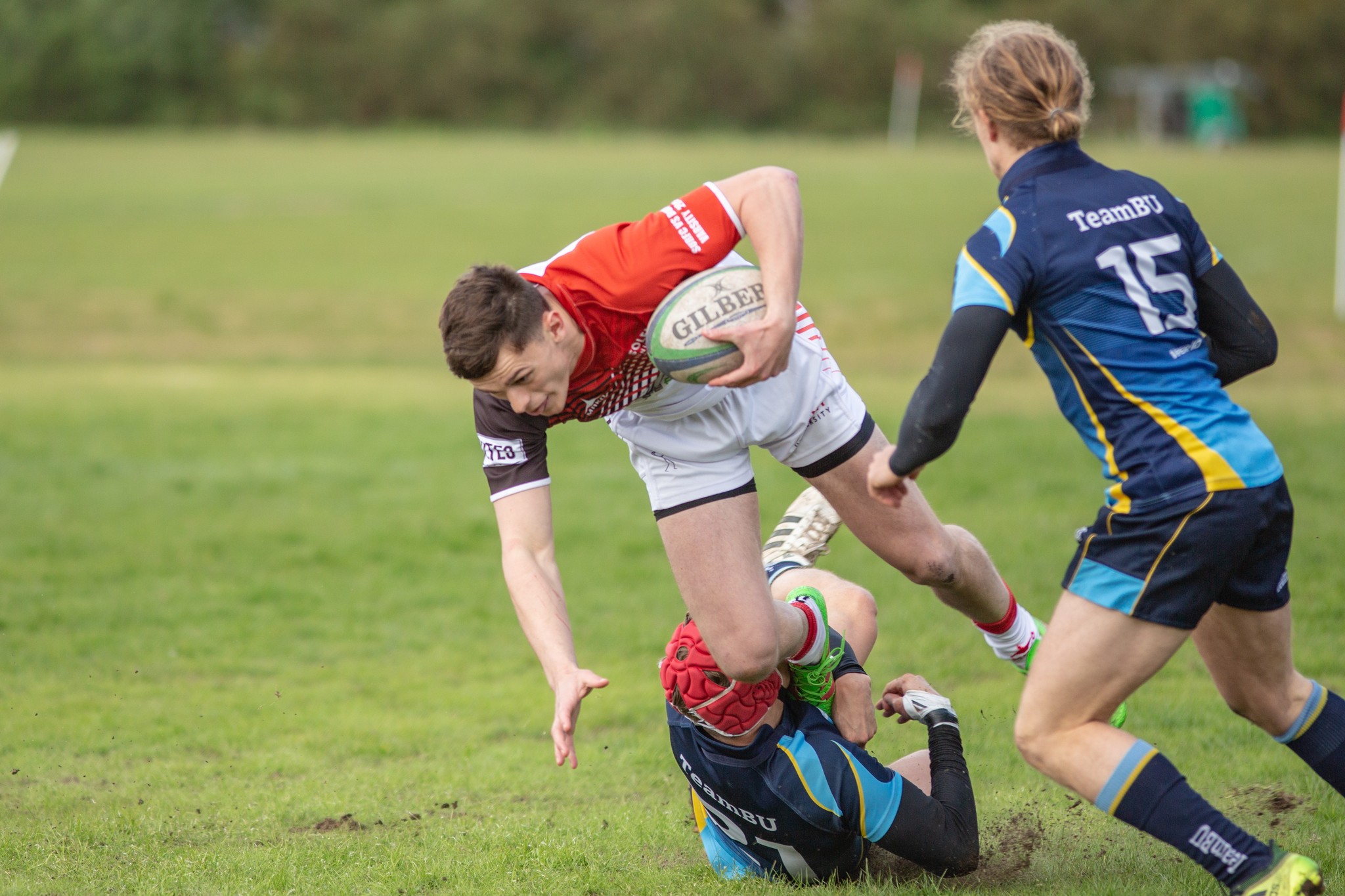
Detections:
[644,264,768,385]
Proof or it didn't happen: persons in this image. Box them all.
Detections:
[658,477,977,886]
[866,20,1345,895]
[435,165,1130,771]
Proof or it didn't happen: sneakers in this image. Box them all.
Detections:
[782,584,848,715]
[1018,615,1128,728]
[762,485,845,574]
[1216,837,1325,896]
